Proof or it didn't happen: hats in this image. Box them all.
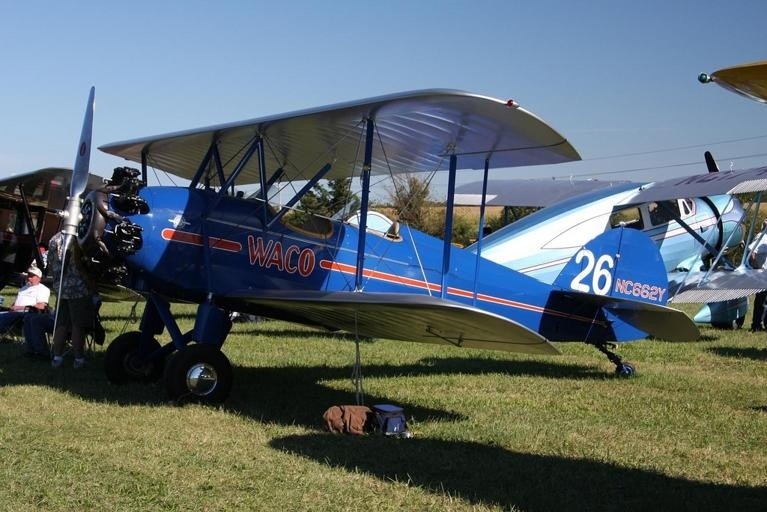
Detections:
[27,266,43,278]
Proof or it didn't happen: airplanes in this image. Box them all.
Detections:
[49,87,703,407]
[442,149,767,307]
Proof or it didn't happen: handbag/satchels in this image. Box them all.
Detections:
[321,404,410,439]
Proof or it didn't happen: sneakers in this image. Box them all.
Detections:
[751,320,765,330]
[51,354,63,368]
[74,356,96,370]
[24,351,40,361]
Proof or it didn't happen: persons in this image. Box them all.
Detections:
[746,219,766,332]
[20,305,62,360]
[45,230,91,369]
[0,265,51,335]
